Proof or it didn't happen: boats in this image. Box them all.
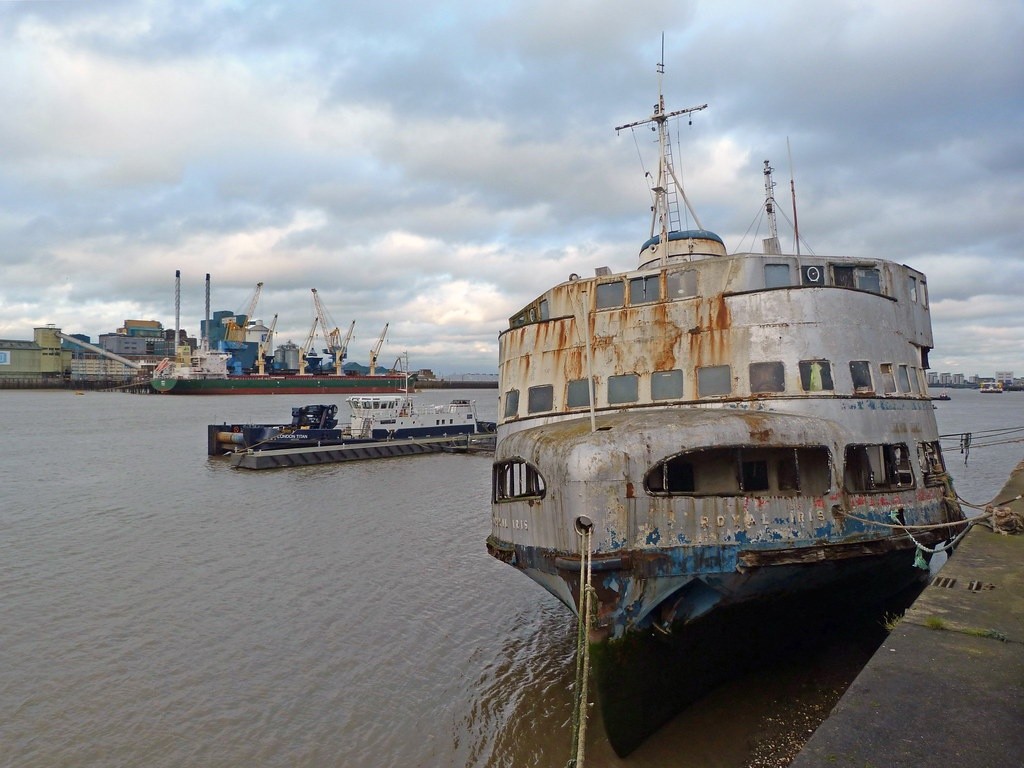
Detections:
[979,382,1003,393]
[347,349,481,439]
[481,28,971,762]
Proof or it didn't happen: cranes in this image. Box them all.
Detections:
[225,280,393,378]
